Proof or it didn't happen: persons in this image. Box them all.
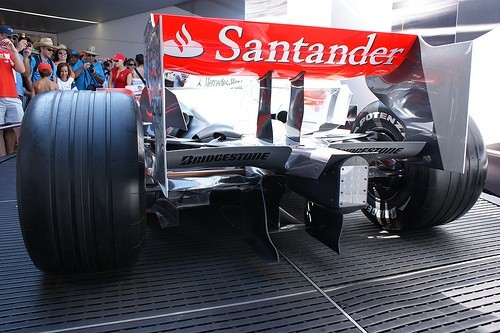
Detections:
[55,63,78,91]
[0,24,32,155]
[25,38,61,92]
[53,44,75,79]
[108,54,133,89]
[130,54,144,79]
[71,46,106,91]
[102,58,114,88]
[0,39,27,159]
[30,62,57,99]
[69,51,78,66]
[19,35,32,56]
[127,58,135,70]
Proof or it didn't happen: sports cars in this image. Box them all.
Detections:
[16,0,500,282]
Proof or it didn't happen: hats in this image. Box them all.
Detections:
[33,38,53,47]
[56,43,67,49]
[111,53,126,60]
[82,47,99,56]
[0,24,17,34]
[38,63,51,73]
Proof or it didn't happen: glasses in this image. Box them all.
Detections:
[59,52,62,54]
[129,63,136,66]
[5,35,11,39]
[114,60,119,62]
[48,48,54,52]
[87,55,95,57]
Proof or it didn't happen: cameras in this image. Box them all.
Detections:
[88,64,95,73]
